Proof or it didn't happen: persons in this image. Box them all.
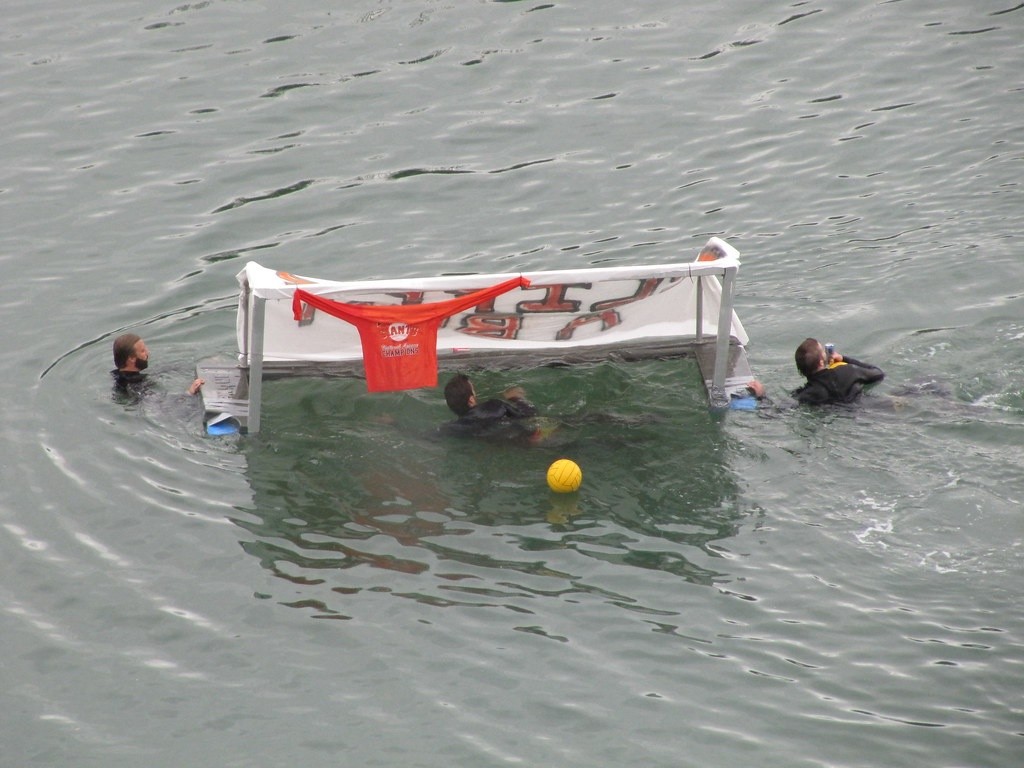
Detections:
[444,375,518,419]
[110,333,205,395]
[747,338,885,401]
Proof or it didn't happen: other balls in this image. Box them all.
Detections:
[547,458,583,494]
